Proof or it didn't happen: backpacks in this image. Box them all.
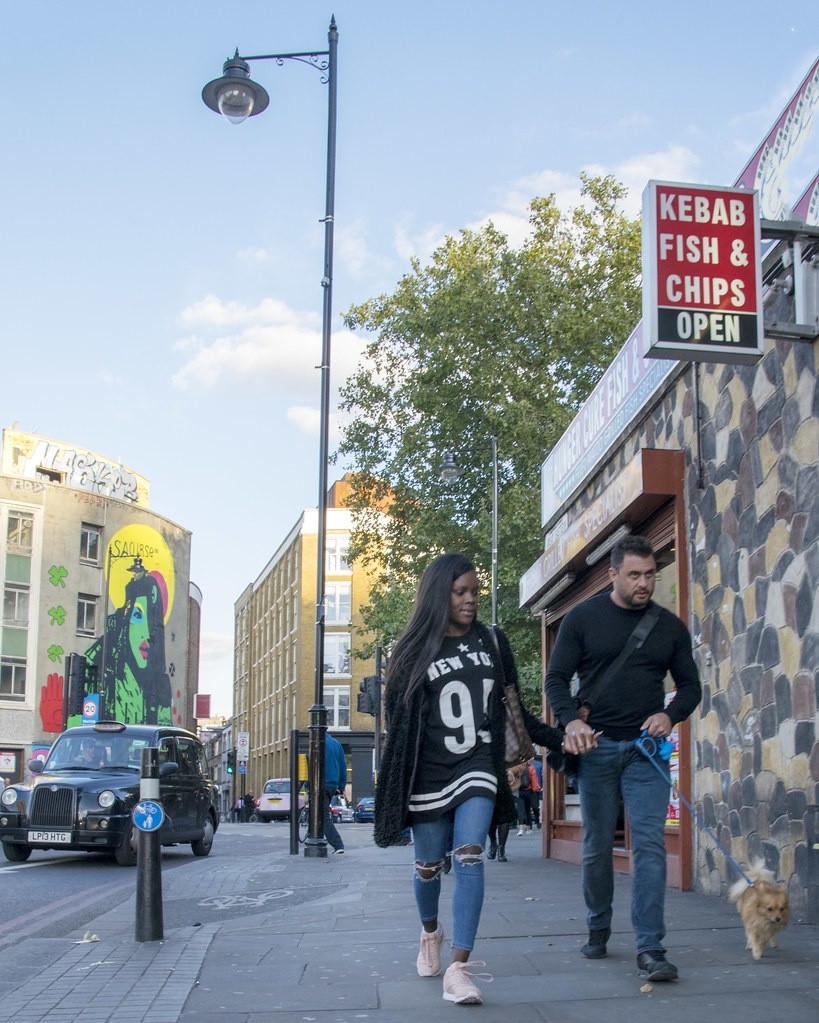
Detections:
[521,765,530,788]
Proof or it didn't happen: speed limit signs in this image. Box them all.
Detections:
[83,693,99,723]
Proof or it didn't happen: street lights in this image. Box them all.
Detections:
[98,544,148,721]
[201,13,339,857]
[438,436,498,627]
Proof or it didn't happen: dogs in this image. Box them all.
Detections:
[729,855,790,961]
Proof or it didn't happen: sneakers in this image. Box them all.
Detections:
[443,960,494,1004]
[637,951,680,981]
[580,926,611,958]
[416,922,445,976]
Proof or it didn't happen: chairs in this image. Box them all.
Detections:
[129,749,141,766]
[95,746,109,764]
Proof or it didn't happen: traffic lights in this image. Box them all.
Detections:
[227,752,234,774]
[357,675,375,715]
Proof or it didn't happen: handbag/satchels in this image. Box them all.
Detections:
[545,696,579,779]
[486,625,537,768]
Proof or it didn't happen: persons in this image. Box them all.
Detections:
[487,750,543,862]
[70,738,106,768]
[5,776,12,788]
[544,537,703,984]
[234,791,256,823]
[373,554,598,1007]
[322,734,347,855]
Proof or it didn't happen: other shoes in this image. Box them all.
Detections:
[517,824,532,836]
[536,823,541,829]
[443,856,451,874]
[332,849,345,854]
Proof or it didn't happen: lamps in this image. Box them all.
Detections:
[530,572,577,615]
[585,524,632,566]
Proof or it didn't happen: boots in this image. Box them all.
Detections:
[498,844,507,862]
[487,840,497,859]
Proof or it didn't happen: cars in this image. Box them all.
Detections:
[352,797,375,823]
[0,721,221,866]
[256,778,309,823]
[329,795,356,822]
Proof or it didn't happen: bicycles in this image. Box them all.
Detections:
[297,790,334,843]
